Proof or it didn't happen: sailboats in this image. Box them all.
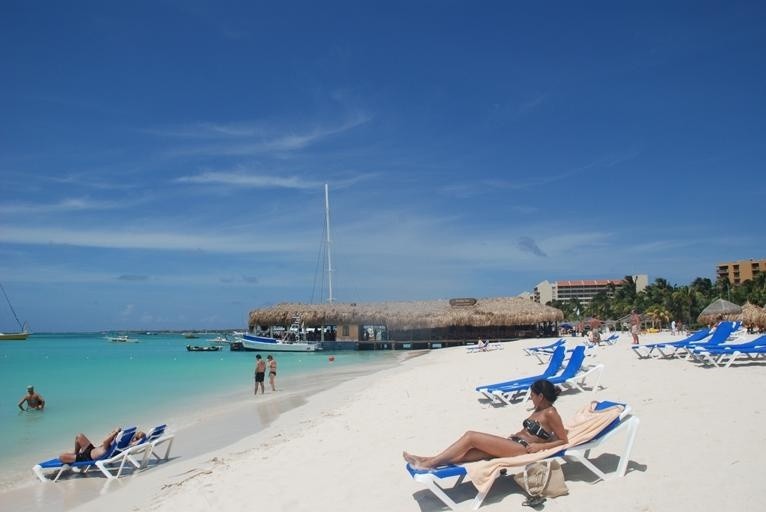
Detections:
[0,267,29,340]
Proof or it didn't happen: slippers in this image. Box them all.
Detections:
[522,496,546,507]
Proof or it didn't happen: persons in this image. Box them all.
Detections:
[58,428,122,465]
[257,326,391,343]
[16,385,45,414]
[555,307,692,345]
[265,354,277,392]
[110,431,147,447]
[252,353,266,395]
[476,334,484,349]
[402,378,569,474]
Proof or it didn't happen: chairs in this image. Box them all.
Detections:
[475,346,567,405]
[584,333,619,346]
[632,321,766,368]
[33,424,176,480]
[406,401,641,511]
[466,340,505,353]
[491,345,604,406]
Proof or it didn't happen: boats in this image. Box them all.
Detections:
[177,332,199,340]
[182,345,224,352]
[104,335,139,344]
[233,329,324,354]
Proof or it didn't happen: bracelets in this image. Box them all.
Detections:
[111,431,117,435]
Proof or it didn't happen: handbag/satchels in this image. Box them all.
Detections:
[514,458,569,498]
